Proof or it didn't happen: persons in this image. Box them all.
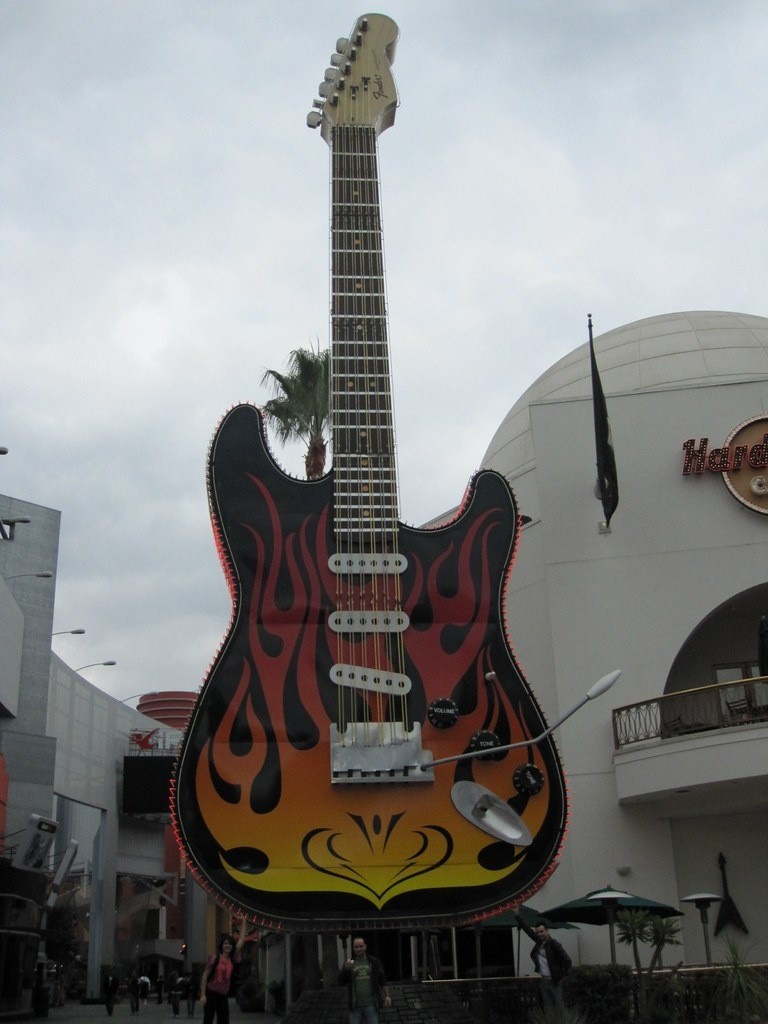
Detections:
[102,966,201,1019]
[338,937,392,1024]
[199,912,246,1024]
[509,903,574,1024]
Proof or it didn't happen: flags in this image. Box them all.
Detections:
[590,345,620,526]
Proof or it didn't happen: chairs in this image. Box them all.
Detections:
[663,716,721,738]
[726,696,768,727]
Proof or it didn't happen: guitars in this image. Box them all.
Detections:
[167,9,571,939]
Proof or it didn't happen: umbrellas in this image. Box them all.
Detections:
[479,904,581,978]
[542,885,683,1024]
[759,616,768,676]
